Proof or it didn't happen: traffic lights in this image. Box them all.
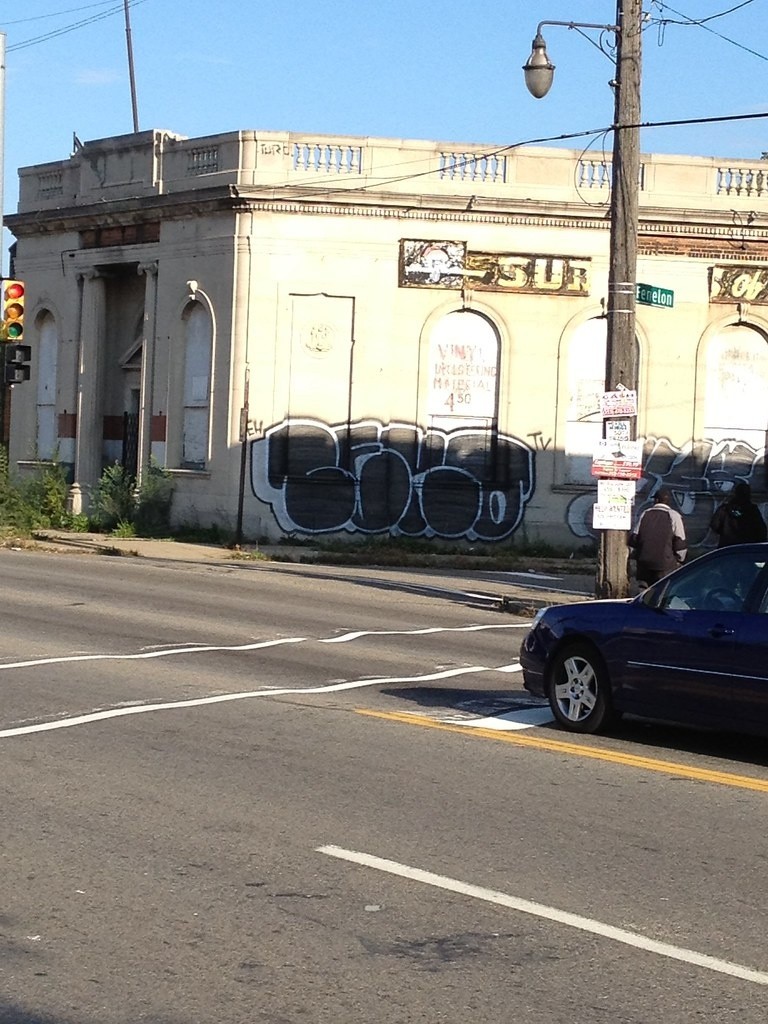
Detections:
[0,342,33,386]
[0,279,25,342]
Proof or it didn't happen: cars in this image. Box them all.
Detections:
[516,539,768,744]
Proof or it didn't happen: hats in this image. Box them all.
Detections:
[736,484,750,494]
[650,488,672,501]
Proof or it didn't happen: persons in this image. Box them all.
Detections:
[710,484,768,598]
[632,489,689,603]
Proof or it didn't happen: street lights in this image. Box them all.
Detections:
[522,19,644,602]
